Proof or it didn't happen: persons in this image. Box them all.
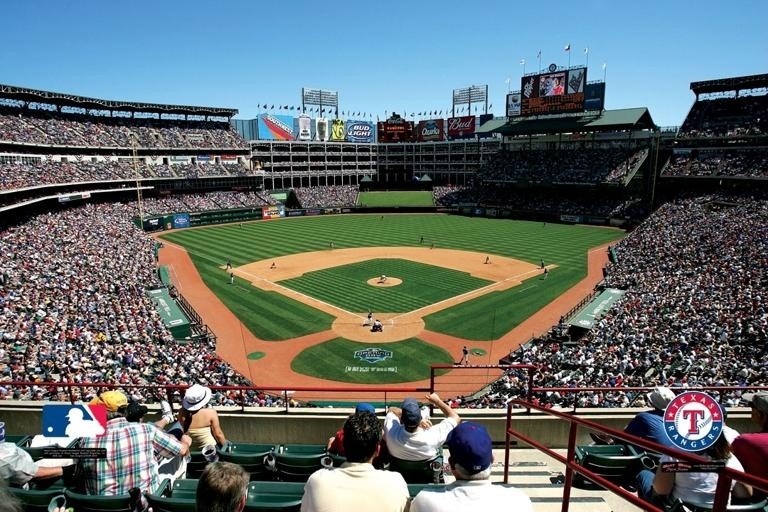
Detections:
[545,77,564,96]
[1,107,767,511]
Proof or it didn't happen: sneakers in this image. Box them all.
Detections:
[589,431,614,444]
[161,400,175,424]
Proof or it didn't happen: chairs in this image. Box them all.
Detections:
[649,485,768,512]
[0,434,447,512]
[549,445,645,490]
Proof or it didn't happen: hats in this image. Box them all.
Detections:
[356,403,375,414]
[182,384,212,412]
[403,398,421,427]
[646,386,676,412]
[446,421,492,470]
[741,391,767,412]
[101,390,128,412]
[126,402,148,420]
